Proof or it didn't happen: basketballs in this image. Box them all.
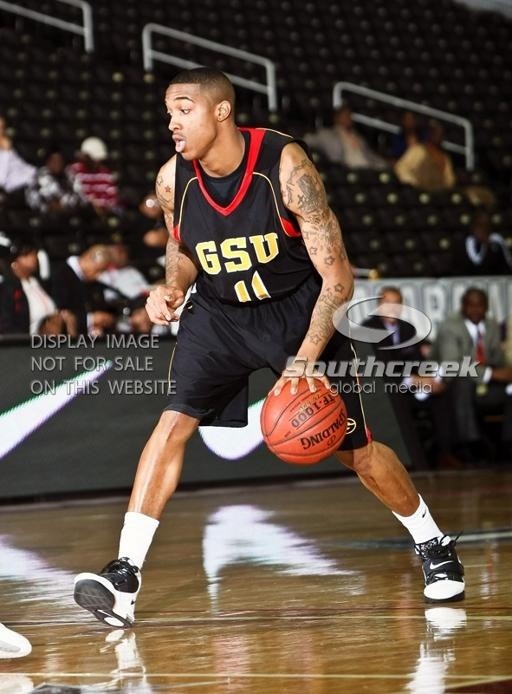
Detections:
[260,377,348,464]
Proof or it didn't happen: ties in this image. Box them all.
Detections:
[476,325,485,367]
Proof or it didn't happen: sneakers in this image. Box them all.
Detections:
[415,531,466,602]
[71,557,140,628]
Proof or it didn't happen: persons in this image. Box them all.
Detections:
[355,286,512,469]
[72,66,465,628]
[393,110,457,192]
[304,106,388,167]
[83,628,458,694]
[0,622,33,658]
[457,210,512,275]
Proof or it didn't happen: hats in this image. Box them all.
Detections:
[81,136,109,161]
